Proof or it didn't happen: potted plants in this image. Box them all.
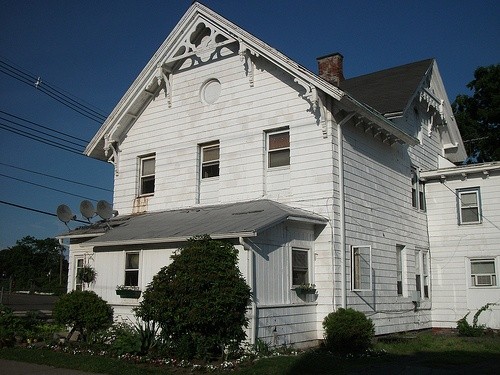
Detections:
[297,282,317,295]
[114,284,142,296]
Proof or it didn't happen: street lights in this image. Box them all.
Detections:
[46,272,51,282]
